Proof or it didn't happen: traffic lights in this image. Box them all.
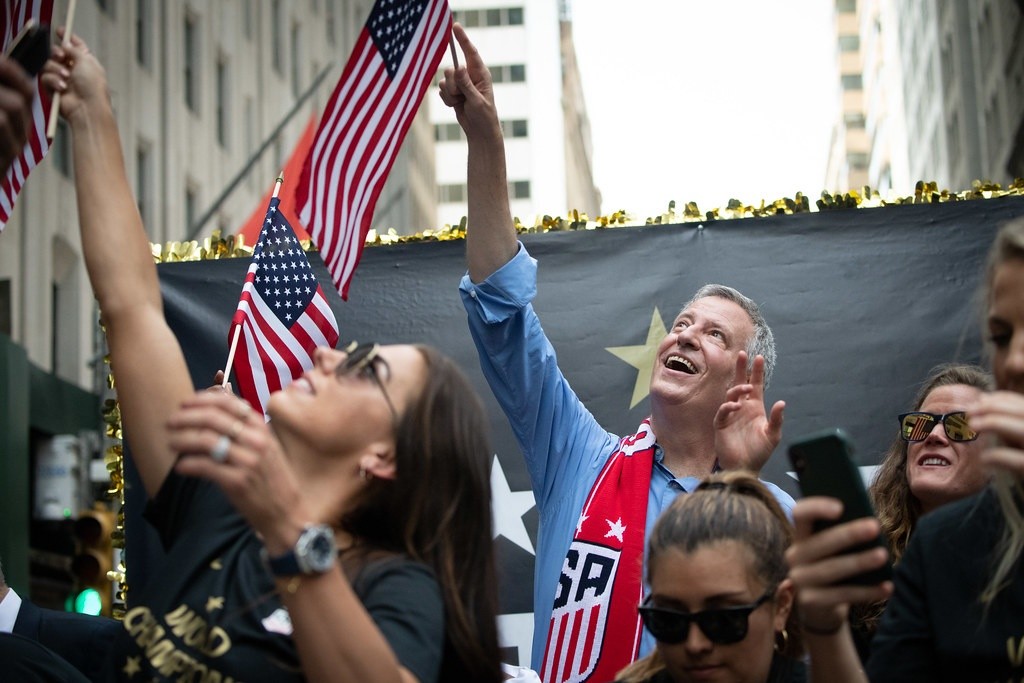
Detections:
[62,510,116,621]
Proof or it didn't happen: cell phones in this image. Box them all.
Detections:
[0,20,50,88]
[786,428,894,586]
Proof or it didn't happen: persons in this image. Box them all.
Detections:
[712,351,991,571]
[437,21,797,683]
[784,219,1024,682]
[0,28,507,683]
[614,470,794,683]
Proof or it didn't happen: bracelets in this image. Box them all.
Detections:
[799,623,844,636]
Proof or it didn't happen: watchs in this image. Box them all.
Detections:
[270,524,338,578]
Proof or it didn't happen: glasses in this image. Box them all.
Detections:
[898,411,979,442]
[637,589,774,646]
[336,340,400,429]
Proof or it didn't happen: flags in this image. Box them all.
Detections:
[0,1,54,234]
[226,197,340,415]
[294,0,454,302]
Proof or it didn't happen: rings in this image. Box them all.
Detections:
[228,422,244,439]
[213,436,231,463]
[235,403,251,420]
